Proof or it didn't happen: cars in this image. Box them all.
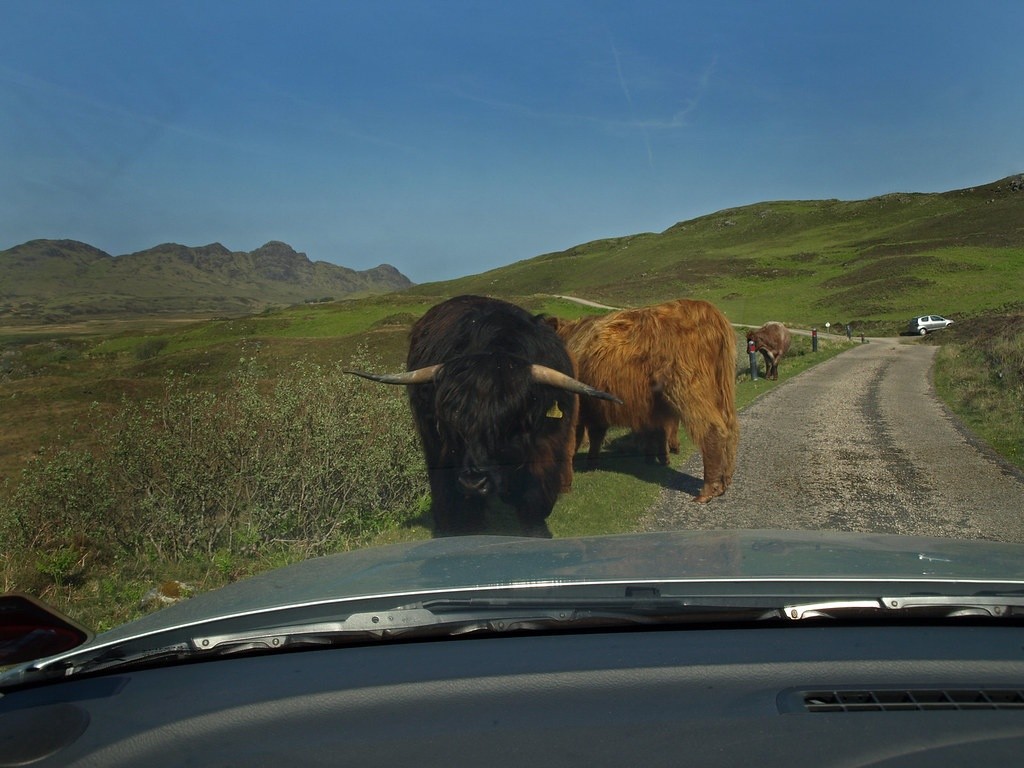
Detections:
[910,314,955,335]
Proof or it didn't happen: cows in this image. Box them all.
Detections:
[343,294,624,539]
[547,299,739,504]
[746,321,792,381]
[638,413,679,465]
